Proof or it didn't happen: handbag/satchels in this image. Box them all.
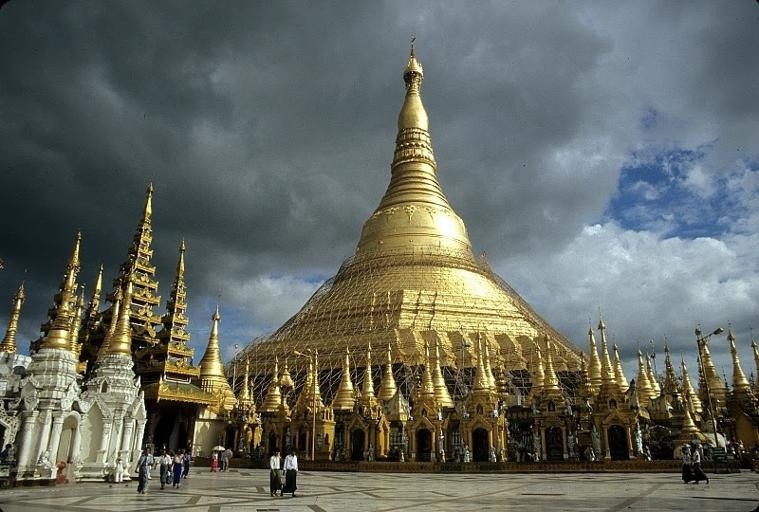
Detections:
[167,474,173,485]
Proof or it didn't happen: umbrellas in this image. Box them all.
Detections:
[212,446,225,452]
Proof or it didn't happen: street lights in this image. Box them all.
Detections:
[293,348,318,463]
[694,327,725,448]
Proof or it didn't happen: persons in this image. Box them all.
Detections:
[154,447,192,491]
[210,449,229,472]
[676,446,694,484]
[689,442,710,484]
[280,446,299,497]
[270,446,283,497]
[701,418,729,454]
[135,446,153,494]
[0,444,12,464]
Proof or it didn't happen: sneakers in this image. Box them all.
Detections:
[160,483,166,490]
[173,475,188,489]
[270,491,299,498]
[136,488,147,495]
